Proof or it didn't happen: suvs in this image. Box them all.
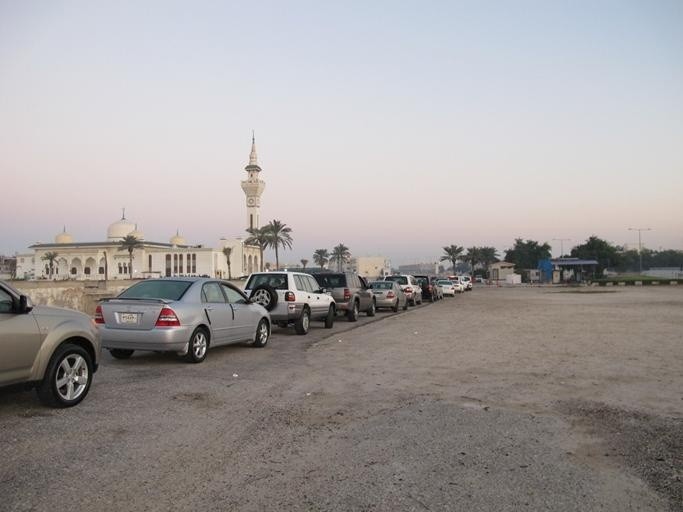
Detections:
[243,271,336,336]
[475,275,481,282]
[314,271,376,322]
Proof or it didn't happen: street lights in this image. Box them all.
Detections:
[627,227,652,273]
[552,238,571,258]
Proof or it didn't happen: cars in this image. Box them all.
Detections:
[95,277,272,363]
[370,274,473,313]
[0,281,101,408]
